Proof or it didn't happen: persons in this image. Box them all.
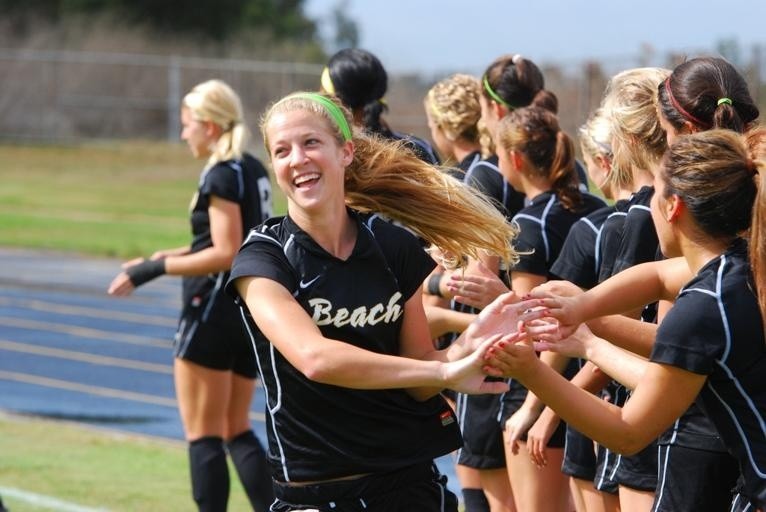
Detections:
[413,50,766,512]
[104,73,284,512]
[219,89,559,512]
[316,45,452,272]
